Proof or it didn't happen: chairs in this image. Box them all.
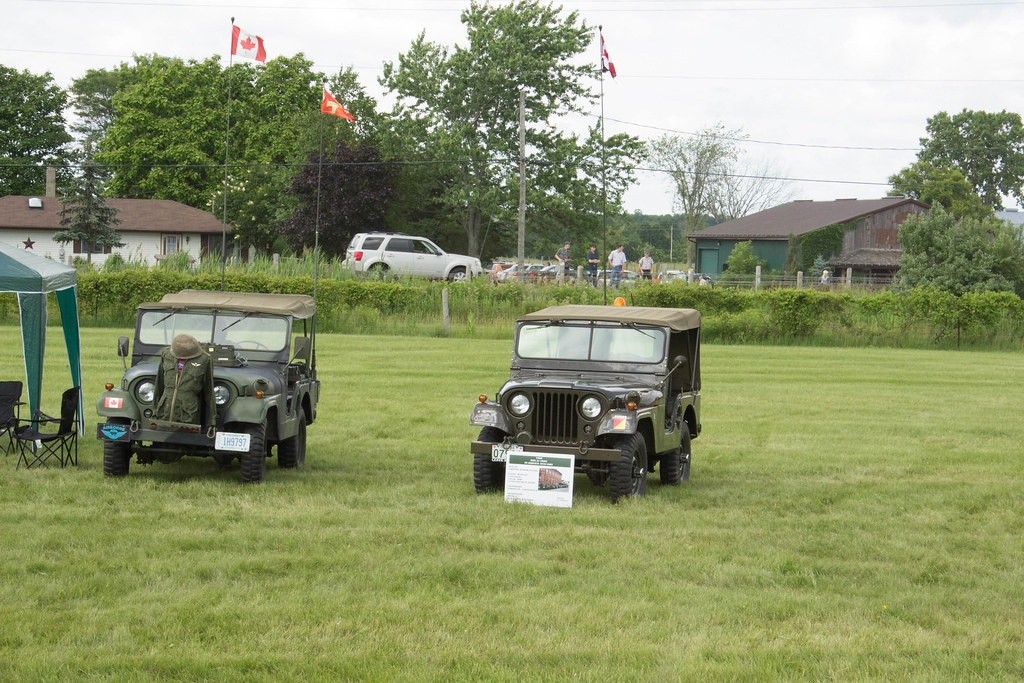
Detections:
[8,386,81,469]
[0,381,27,456]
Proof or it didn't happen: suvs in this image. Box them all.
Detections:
[95,290,321,486]
[470,297,702,506]
[341,230,483,284]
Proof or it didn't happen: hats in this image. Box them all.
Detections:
[170,334,202,359]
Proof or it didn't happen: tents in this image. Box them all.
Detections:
[0,240,84,454]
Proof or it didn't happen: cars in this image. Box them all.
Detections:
[491,261,714,290]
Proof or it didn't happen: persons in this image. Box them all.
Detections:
[555,241,654,290]
[822,270,830,284]
[153,335,217,427]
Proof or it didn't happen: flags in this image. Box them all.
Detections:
[321,89,354,123]
[600,37,617,78]
[233,24,267,64]
